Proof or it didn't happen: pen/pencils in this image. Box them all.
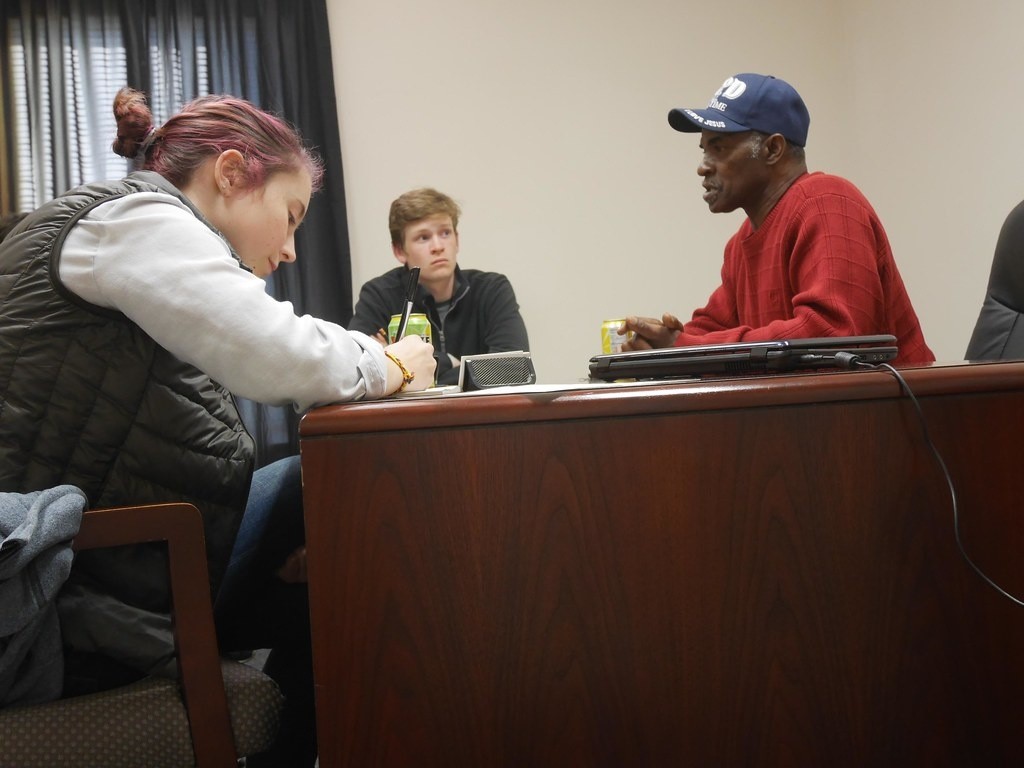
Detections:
[393,266,421,343]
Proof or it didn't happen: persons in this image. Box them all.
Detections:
[349,187,537,389]
[1,86,439,768]
[618,71,937,370]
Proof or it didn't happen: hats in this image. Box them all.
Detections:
[668,72,810,147]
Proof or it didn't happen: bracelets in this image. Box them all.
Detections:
[382,349,415,396]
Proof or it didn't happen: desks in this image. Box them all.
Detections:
[296,362,1024,768]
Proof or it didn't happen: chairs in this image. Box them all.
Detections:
[0,503,288,768]
[963,200,1024,363]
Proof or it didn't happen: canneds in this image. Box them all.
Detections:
[601,319,636,382]
[388,314,432,345]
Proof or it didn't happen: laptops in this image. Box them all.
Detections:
[589,334,897,383]
